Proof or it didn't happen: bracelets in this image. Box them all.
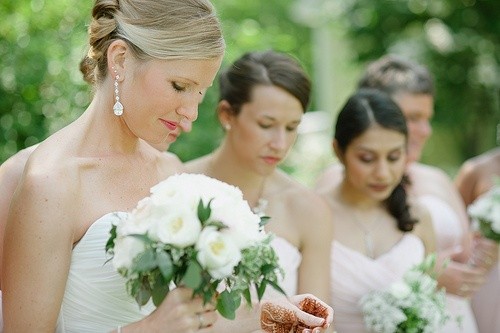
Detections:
[116,327,121,333]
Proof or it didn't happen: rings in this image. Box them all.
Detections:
[199,313,204,328]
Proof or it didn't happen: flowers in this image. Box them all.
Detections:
[467,174,500,270]
[102,173,290,321]
[358,252,451,333]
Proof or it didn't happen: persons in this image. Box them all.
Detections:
[455,150,500,333]
[0,0,334,333]
[182,49,333,333]
[315,52,500,333]
[312,89,439,333]
